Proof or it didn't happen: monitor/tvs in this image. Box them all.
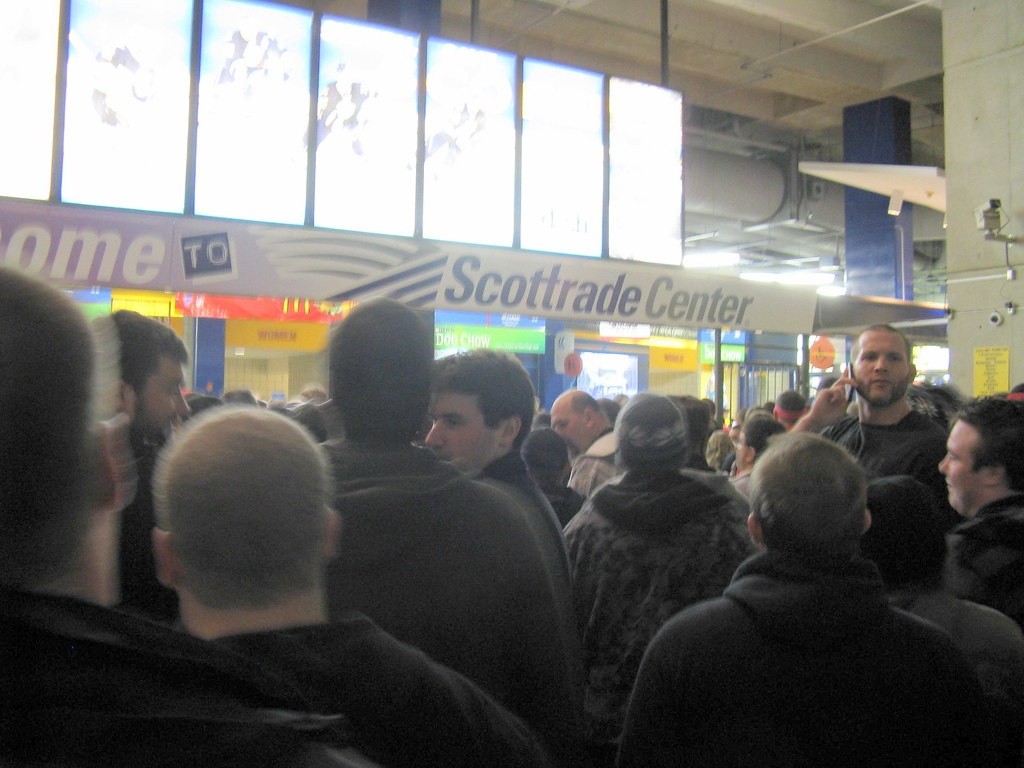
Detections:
[699,328,750,364]
[434,310,549,359]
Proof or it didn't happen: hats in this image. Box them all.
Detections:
[617,395,689,475]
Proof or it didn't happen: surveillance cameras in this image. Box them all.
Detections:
[974,199,1001,213]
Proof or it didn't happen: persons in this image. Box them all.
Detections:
[1,264,1024,768]
[616,430,999,768]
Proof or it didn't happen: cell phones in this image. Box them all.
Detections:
[842,362,855,403]
